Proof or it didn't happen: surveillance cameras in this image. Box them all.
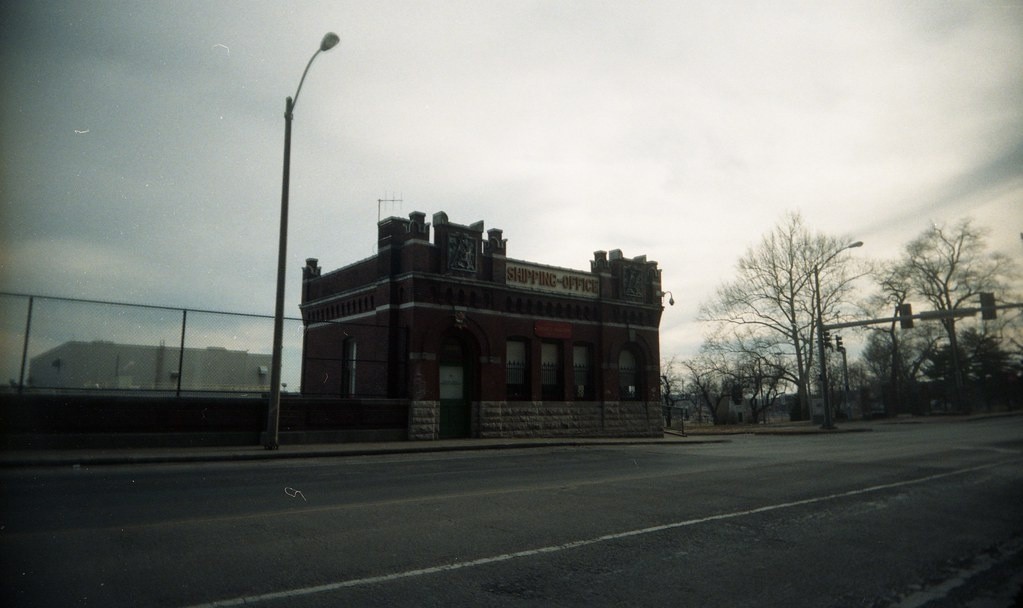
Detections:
[669,298,674,305]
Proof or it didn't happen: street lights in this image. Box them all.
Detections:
[813,239,864,428]
[262,30,341,452]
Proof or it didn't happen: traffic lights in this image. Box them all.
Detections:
[836,335,843,351]
[823,331,830,349]
